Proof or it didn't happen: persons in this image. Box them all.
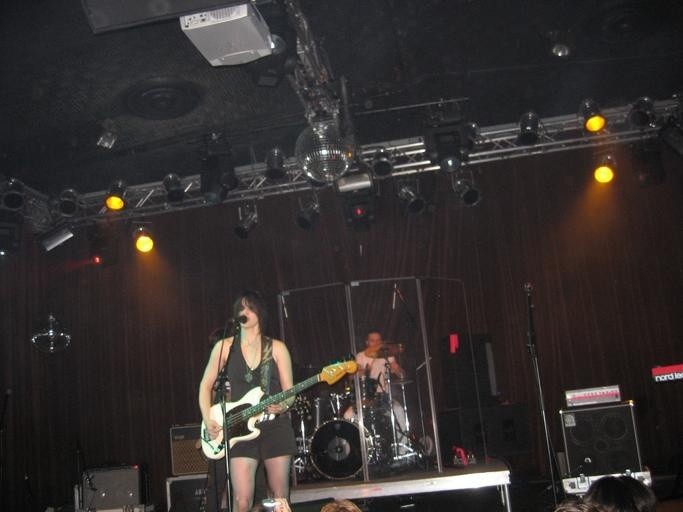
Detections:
[343,328,407,456]
[548,473,683,512]
[320,498,362,512]
[198,290,296,512]
[201,326,268,511]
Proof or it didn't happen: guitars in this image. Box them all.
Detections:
[201,360,358,460]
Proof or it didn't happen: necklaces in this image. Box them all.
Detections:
[242,349,256,382]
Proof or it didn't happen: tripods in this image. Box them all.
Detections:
[383,380,434,472]
[294,415,318,483]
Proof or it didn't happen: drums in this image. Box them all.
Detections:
[308,417,375,481]
[350,390,392,417]
[351,377,378,393]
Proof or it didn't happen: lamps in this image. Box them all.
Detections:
[592,146,625,185]
[264,141,286,176]
[56,186,85,215]
[129,220,158,256]
[233,200,259,237]
[629,94,659,127]
[396,177,425,214]
[625,142,666,190]
[574,96,607,133]
[425,100,482,206]
[104,175,128,211]
[373,144,395,180]
[296,94,357,185]
[162,171,183,202]
[520,109,549,147]
[342,190,374,223]
[34,221,74,252]
[1,177,25,209]
[297,190,321,229]
[78,235,110,270]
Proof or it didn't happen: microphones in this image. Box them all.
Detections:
[384,346,389,359]
[227,315,247,326]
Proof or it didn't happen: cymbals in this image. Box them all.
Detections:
[391,378,415,386]
[364,342,406,358]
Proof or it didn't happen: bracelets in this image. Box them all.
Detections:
[282,399,290,412]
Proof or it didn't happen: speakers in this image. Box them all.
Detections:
[560,402,644,477]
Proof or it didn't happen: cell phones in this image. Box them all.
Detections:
[261,498,276,512]
